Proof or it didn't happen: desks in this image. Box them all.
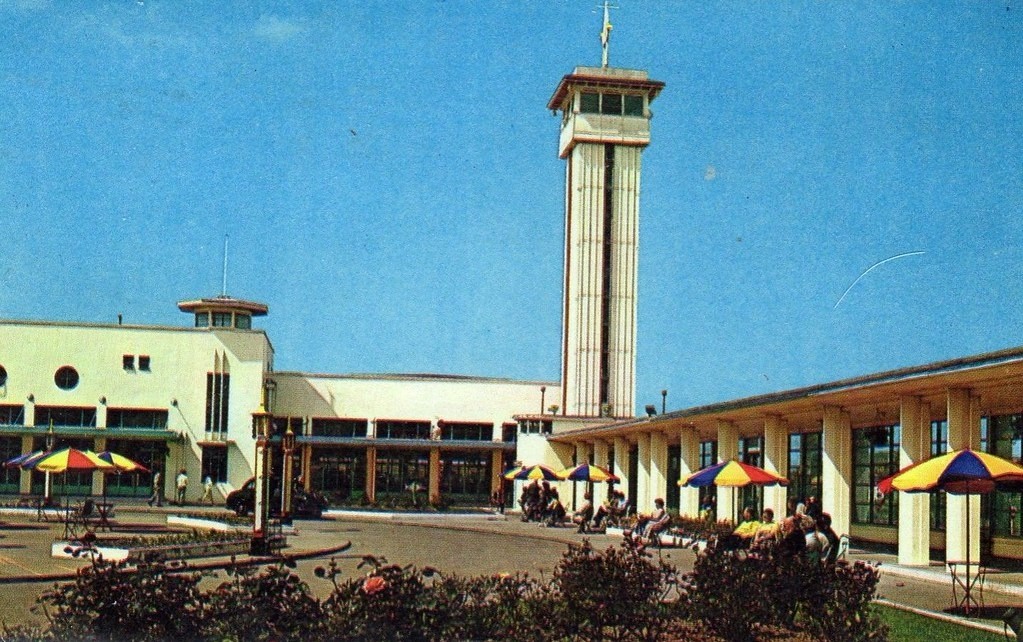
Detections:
[934,559,991,618]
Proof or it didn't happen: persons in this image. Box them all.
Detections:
[199,470,217,507]
[728,505,759,552]
[750,509,780,554]
[175,468,189,505]
[772,493,840,576]
[698,493,717,526]
[641,498,666,540]
[571,492,593,528]
[145,465,165,507]
[594,488,627,530]
[485,479,565,528]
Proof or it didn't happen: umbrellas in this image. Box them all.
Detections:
[880,447,1023,618]
[673,457,792,536]
[503,463,566,486]
[2,443,152,540]
[555,458,620,528]
